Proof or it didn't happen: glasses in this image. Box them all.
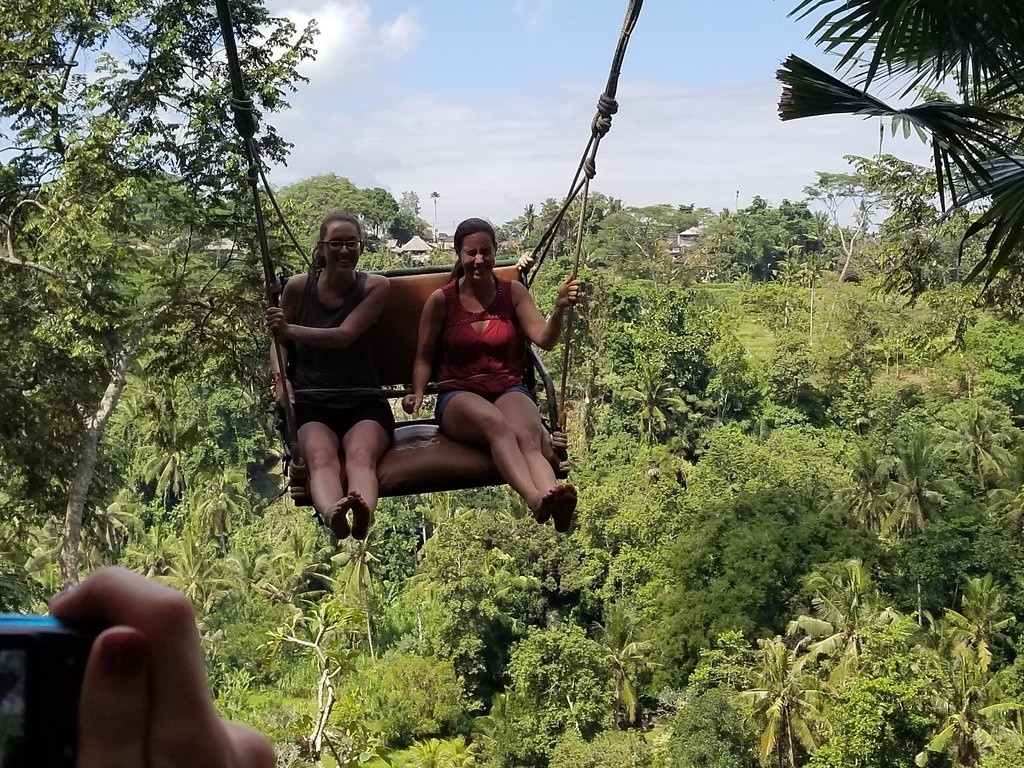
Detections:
[320,240,361,251]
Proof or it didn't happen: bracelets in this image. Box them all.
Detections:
[275,372,287,384]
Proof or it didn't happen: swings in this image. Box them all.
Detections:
[214,0,643,507]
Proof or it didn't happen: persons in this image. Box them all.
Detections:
[54,564,280,768]
[402,218,579,534]
[269,211,392,540]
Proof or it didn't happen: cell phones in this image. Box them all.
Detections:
[2,616,108,768]
